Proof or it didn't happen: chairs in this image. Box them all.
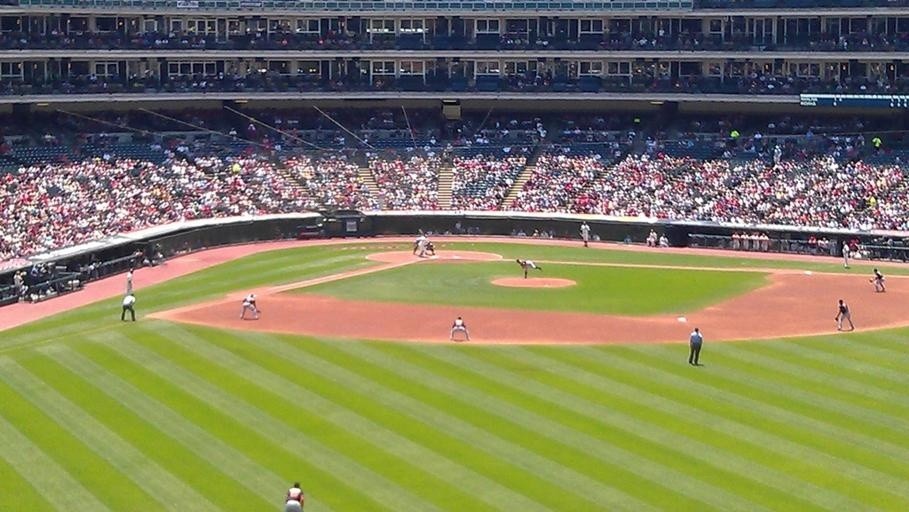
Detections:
[363,134,635,169]
[647,135,904,167]
[0,134,347,180]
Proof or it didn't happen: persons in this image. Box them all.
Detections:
[450,315,469,341]
[835,299,856,331]
[241,293,262,322]
[285,482,306,512]
[687,326,703,366]
[120,294,136,322]
